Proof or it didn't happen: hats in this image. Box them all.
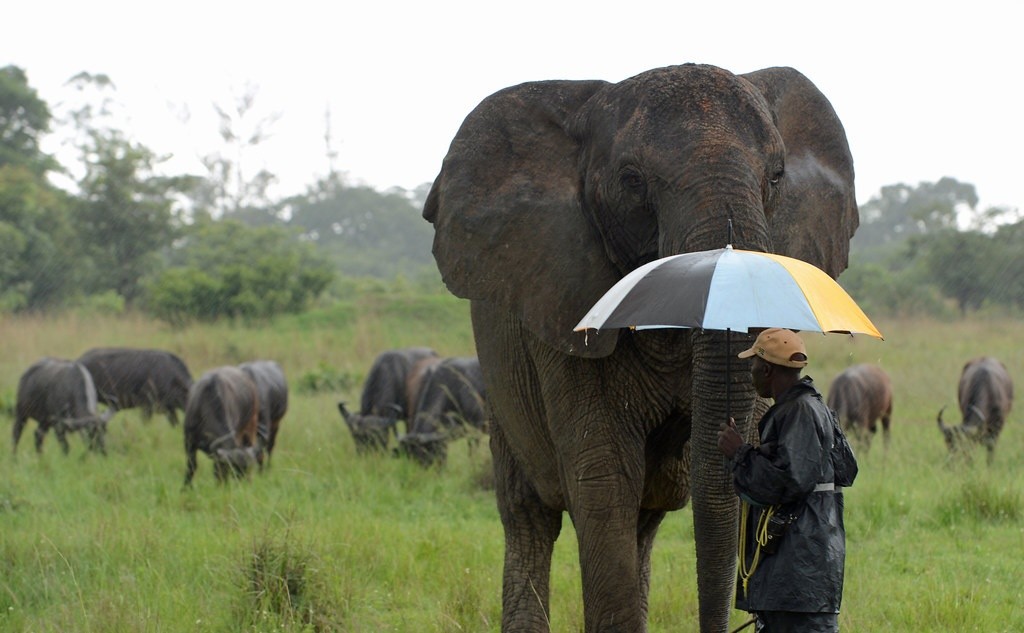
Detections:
[737,328,808,367]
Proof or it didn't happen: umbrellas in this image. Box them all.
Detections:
[571,244,886,425]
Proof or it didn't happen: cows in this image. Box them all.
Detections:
[825,364,893,459]
[9,344,289,492]
[336,347,492,471]
[936,354,1014,468]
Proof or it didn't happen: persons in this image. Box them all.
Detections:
[718,328,858,633]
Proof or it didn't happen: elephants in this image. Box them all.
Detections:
[419,61,863,633]
